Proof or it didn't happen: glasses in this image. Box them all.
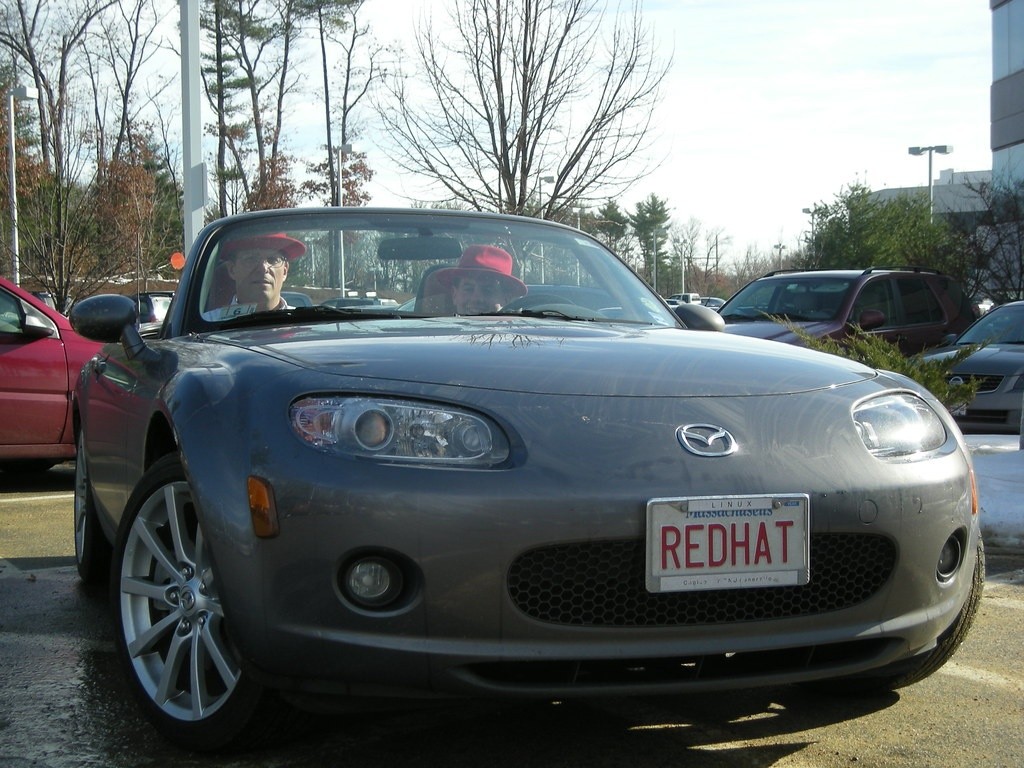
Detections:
[232,251,288,268]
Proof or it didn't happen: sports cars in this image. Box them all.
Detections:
[68,203,988,756]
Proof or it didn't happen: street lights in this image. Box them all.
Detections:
[336,143,353,298]
[774,243,787,272]
[802,207,823,257]
[653,228,660,293]
[7,85,39,288]
[680,239,688,294]
[908,145,954,223]
[572,207,582,286]
[538,176,556,285]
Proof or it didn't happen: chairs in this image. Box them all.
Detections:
[414,264,457,312]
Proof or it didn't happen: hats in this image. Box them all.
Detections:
[437,244,528,297]
[219,229,307,260]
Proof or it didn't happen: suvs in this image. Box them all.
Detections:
[714,265,981,369]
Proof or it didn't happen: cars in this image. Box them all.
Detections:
[111,270,164,285]
[279,290,314,307]
[659,291,725,315]
[0,277,110,475]
[904,297,1024,435]
[318,286,620,313]
[129,290,175,330]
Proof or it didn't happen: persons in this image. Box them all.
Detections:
[194,231,307,324]
[431,244,526,315]
